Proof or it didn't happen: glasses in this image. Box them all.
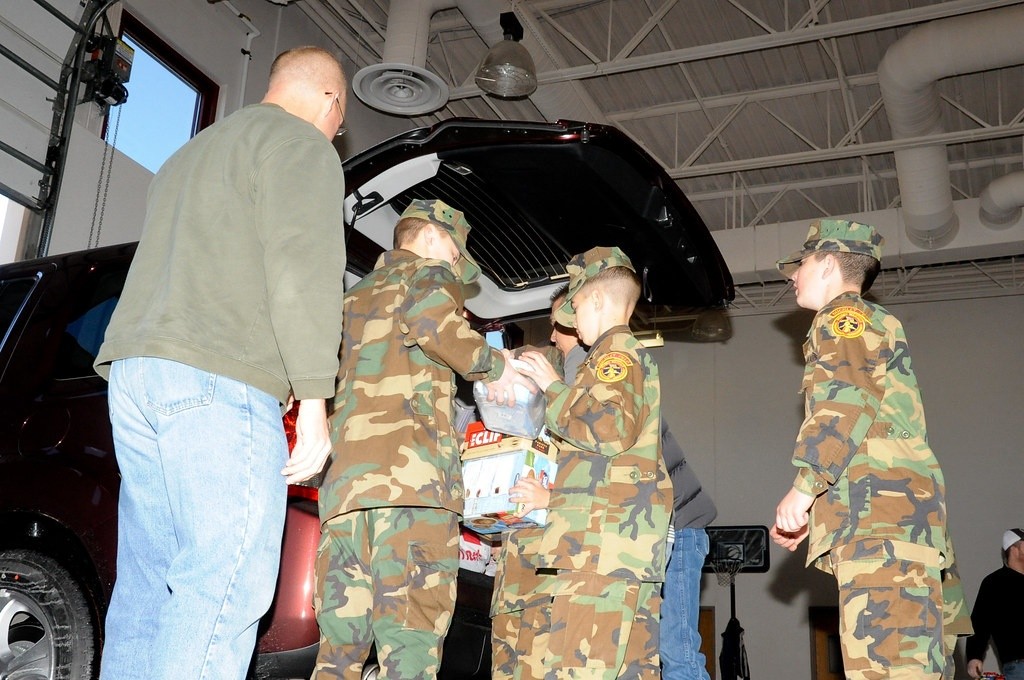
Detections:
[326,91,348,136]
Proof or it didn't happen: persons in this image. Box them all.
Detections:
[310,198,534,680]
[491,281,717,680]
[522,246,675,680]
[967,528,1024,680]
[93,44,342,680]
[768,218,947,679]
[940,528,975,680]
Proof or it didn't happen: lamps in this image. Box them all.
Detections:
[476,11,537,97]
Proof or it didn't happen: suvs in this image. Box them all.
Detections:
[0,115,737,680]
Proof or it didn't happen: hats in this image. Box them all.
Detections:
[398,198,482,285]
[1003,528,1023,550]
[776,219,883,279]
[555,246,637,327]
